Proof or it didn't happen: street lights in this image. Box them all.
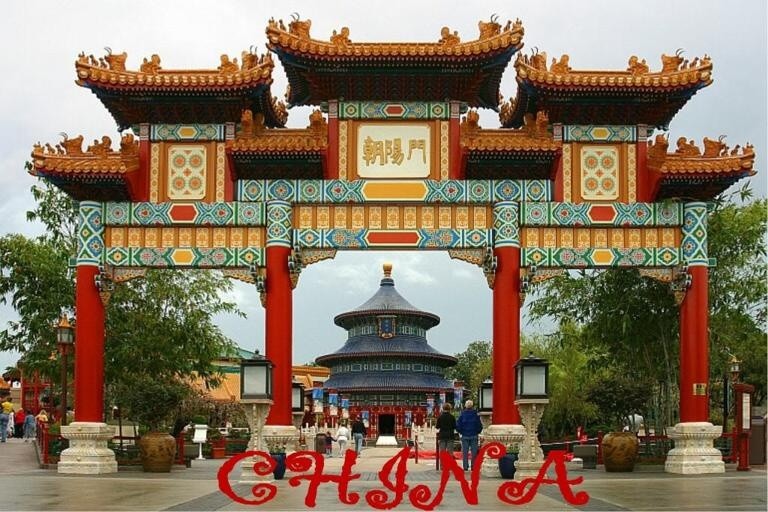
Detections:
[17,357,26,411]
[727,353,743,465]
[54,313,76,467]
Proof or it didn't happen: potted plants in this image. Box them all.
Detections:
[583,364,660,473]
[264,437,292,480]
[499,442,520,479]
[105,374,194,473]
[209,429,226,458]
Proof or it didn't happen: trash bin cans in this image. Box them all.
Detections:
[749,416,764,464]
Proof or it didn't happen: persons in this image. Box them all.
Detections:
[0,396,48,444]
[435,402,457,470]
[335,423,350,458]
[455,399,484,474]
[325,431,337,459]
[351,415,367,458]
